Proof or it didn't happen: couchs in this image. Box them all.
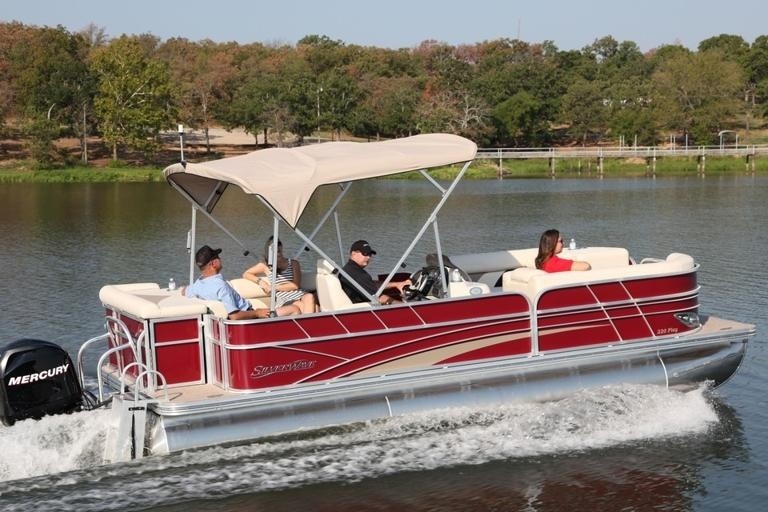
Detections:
[103,280,228,322]
[233,271,322,312]
[503,250,696,300]
[448,245,633,286]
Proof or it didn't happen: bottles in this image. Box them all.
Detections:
[569,238,576,249]
[452,269,460,283]
[168,278,176,291]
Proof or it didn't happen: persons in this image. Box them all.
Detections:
[182,245,302,322]
[242,236,321,317]
[338,240,413,305]
[535,228,591,272]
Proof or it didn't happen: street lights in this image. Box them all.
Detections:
[317,87,323,143]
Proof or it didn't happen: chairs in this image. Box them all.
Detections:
[314,259,372,312]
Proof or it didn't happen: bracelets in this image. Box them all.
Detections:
[256,279,263,285]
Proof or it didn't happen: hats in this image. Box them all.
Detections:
[195,245,222,267]
[352,240,376,255]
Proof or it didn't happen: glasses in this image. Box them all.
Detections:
[558,240,563,243]
[362,252,372,257]
[277,241,281,246]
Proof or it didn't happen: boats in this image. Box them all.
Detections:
[0,134,755,464]
